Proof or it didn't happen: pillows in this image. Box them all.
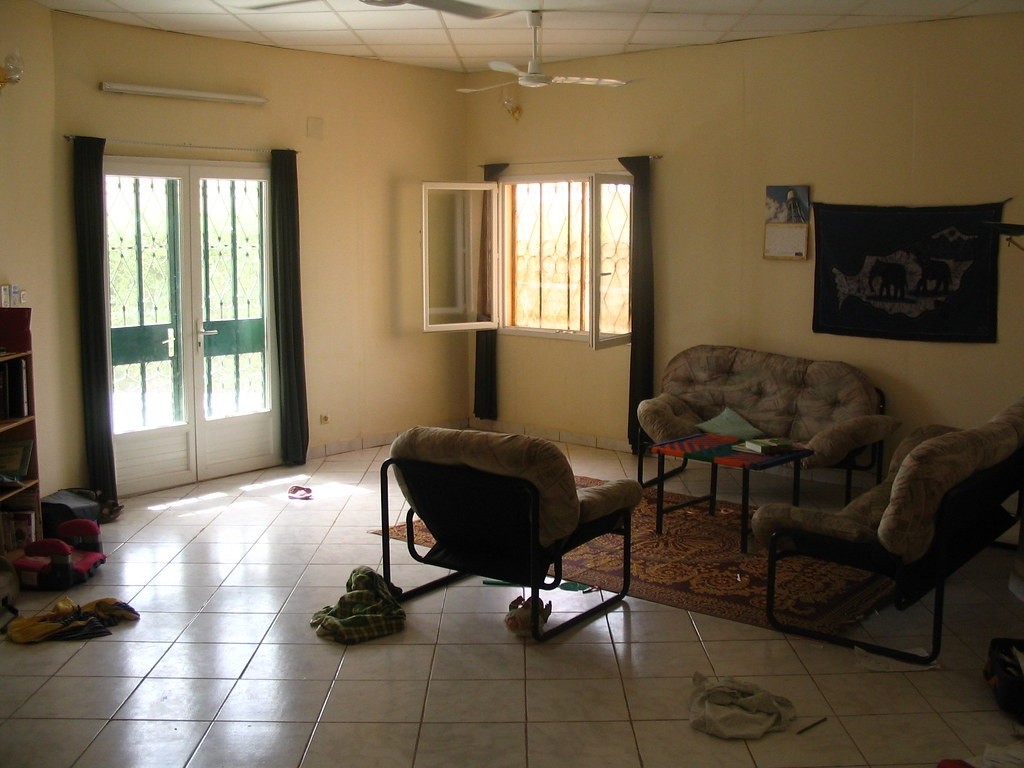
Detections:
[805,414,895,469]
[695,407,766,441]
[637,393,704,444]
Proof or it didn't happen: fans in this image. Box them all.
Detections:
[456,11,627,93]
[238,0,515,19]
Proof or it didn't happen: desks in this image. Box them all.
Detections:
[651,433,815,553]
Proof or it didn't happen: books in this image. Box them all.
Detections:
[0,357,33,478]
[732,436,794,454]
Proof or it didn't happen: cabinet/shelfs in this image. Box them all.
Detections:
[0,306,44,577]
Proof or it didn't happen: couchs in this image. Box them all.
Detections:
[752,396,1024,665]
[380,426,642,644]
[637,344,898,509]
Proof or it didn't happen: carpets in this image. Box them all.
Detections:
[372,475,903,646]
